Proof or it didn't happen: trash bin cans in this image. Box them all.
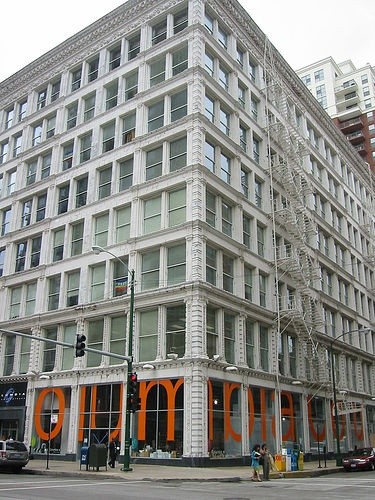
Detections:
[86,444,107,472]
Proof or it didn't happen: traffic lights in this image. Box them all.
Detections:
[74,333,86,359]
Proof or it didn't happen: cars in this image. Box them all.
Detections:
[340,448,375,470]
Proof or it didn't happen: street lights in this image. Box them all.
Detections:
[92,244,137,471]
[328,328,370,466]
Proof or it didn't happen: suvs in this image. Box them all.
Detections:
[0,439,29,471]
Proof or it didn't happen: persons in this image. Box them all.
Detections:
[251,445,261,481]
[259,444,272,481]
[8,435,13,439]
[108,437,118,469]
